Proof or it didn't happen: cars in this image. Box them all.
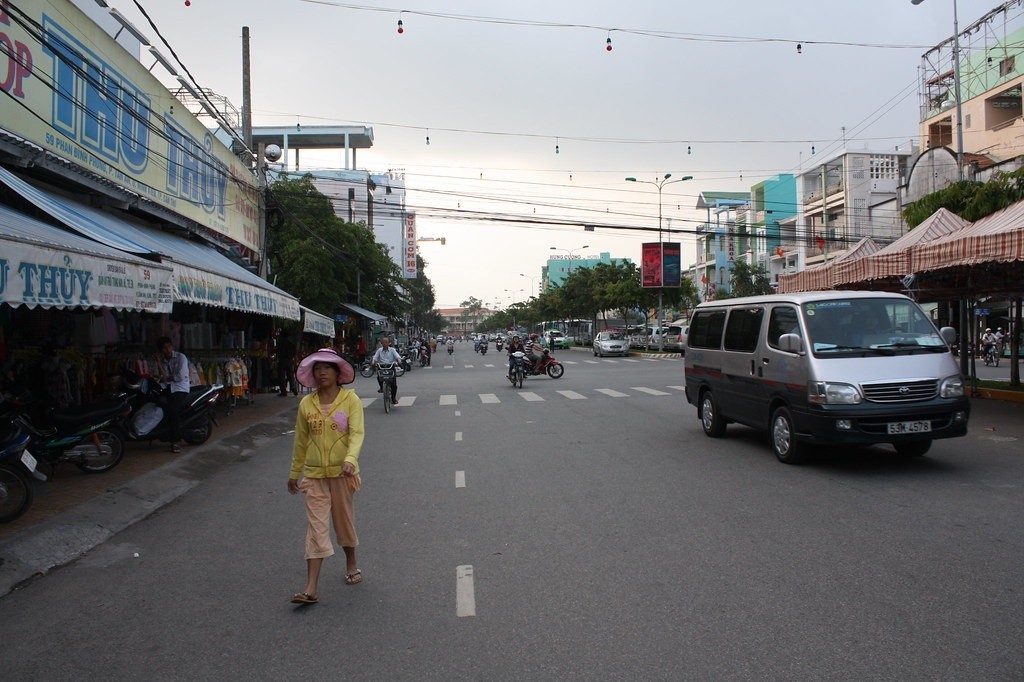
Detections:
[436,335,444,343]
[593,332,630,357]
[544,331,569,350]
[470,330,528,344]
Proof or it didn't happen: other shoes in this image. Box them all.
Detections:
[377,388,383,393]
[391,399,399,404]
[292,386,298,395]
[508,373,512,379]
[280,391,287,396]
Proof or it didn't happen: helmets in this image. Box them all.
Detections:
[513,334,520,342]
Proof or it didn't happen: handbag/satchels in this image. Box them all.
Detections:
[132,401,164,436]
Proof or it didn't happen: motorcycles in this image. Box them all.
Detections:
[111,374,225,451]
[504,346,528,388]
[0,421,48,526]
[980,342,1000,367]
[522,348,565,378]
[0,389,135,474]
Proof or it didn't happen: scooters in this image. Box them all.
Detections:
[448,343,454,355]
[495,339,503,352]
[359,345,431,378]
[474,340,488,356]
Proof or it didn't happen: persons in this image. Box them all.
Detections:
[356,333,366,366]
[982,328,998,358]
[288,348,365,602]
[276,332,298,397]
[446,337,454,344]
[852,311,880,345]
[474,335,488,350]
[539,331,556,354]
[407,336,436,365]
[496,334,503,349]
[152,337,190,453]
[376,334,397,353]
[526,333,544,375]
[371,336,401,404]
[0,357,49,389]
[996,327,1006,357]
[506,336,528,379]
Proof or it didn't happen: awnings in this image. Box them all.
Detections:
[888,297,991,321]
[339,302,388,321]
[0,163,336,339]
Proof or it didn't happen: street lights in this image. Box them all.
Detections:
[519,273,543,332]
[505,289,524,331]
[550,245,589,338]
[625,173,693,354]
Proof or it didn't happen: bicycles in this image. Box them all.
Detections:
[371,361,402,414]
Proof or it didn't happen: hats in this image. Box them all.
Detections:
[297,348,356,387]
[530,333,538,338]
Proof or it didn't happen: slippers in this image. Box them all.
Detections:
[291,592,320,603]
[171,443,180,454]
[345,568,361,584]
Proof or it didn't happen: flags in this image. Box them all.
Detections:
[701,275,706,283]
[777,248,783,257]
[816,236,826,252]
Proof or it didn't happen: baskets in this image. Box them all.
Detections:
[378,369,395,378]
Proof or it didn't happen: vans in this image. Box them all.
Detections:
[685,291,971,466]
[602,323,690,355]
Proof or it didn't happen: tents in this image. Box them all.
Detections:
[778,198,1024,296]
